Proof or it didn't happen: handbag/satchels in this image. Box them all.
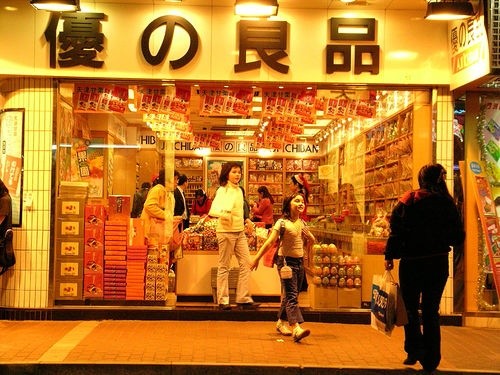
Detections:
[389,272,408,326]
[263,221,283,267]
[371,270,398,337]
[148,216,183,251]
[239,185,249,218]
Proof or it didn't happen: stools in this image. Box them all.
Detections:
[211,267,239,303]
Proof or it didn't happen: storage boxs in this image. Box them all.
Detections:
[308,282,338,310]
[55,182,169,302]
[336,287,361,309]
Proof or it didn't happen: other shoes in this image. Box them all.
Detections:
[220,303,231,309]
[237,301,259,308]
[404,351,418,366]
[276,324,292,335]
[293,329,310,342]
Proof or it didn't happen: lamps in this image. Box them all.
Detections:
[233,0,279,17]
[424,0,476,21]
[29,0,81,12]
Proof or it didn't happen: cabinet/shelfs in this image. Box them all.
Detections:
[174,156,205,207]
[284,155,324,223]
[245,156,284,219]
[365,103,414,236]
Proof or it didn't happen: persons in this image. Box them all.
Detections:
[208,162,262,311]
[322,183,363,223]
[239,185,250,219]
[191,188,212,218]
[252,185,275,229]
[130,168,191,240]
[0,178,16,275]
[289,173,312,221]
[249,194,316,342]
[383,165,465,370]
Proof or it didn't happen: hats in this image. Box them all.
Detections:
[295,174,306,185]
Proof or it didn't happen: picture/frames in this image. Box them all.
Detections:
[0,107,25,229]
